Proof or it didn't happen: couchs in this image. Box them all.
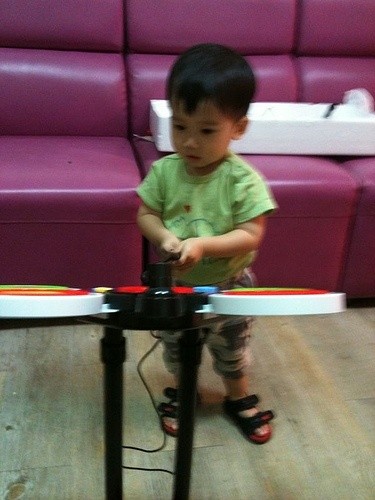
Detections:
[0,0,375,303]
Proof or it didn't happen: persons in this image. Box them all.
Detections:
[136,41,275,444]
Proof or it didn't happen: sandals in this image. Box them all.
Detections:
[156,387,201,435]
[221,391,274,444]
